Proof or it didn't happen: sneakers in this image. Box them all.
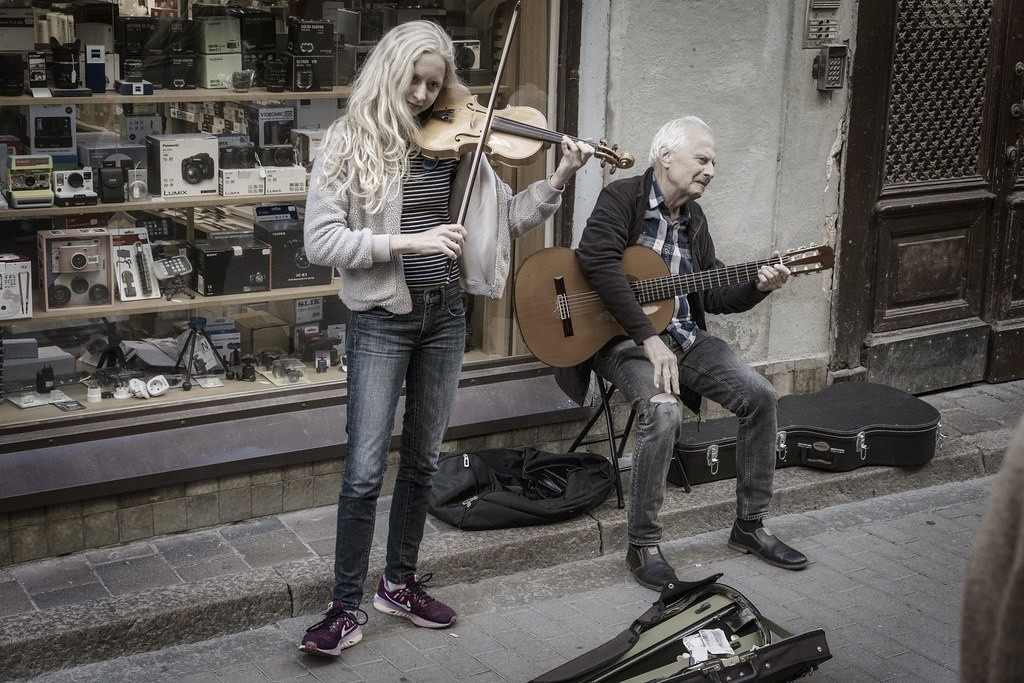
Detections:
[298,599,369,657]
[373,573,457,630]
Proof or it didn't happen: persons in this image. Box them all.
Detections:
[552,115,809,591]
[299,18,595,659]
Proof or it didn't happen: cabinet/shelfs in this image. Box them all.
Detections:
[0,0,522,429]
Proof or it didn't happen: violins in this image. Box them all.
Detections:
[411,83,635,175]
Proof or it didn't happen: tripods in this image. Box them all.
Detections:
[169,317,235,390]
[166,275,196,300]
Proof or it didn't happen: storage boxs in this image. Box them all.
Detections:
[0,98,351,199]
[0,0,490,96]
[0,202,346,395]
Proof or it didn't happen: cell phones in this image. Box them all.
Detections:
[150,254,192,280]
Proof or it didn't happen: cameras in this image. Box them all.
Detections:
[181,152,214,184]
[52,169,99,206]
[63,244,100,272]
[249,272,265,287]
[220,145,256,170]
[35,116,71,136]
[259,144,294,166]
[5,154,54,207]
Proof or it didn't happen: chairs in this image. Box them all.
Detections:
[564,361,693,509]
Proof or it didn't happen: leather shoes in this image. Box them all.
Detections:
[625,542,679,592]
[728,520,808,569]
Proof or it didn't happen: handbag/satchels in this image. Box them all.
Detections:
[427,447,616,531]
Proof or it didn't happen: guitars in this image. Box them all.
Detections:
[512,244,835,368]
